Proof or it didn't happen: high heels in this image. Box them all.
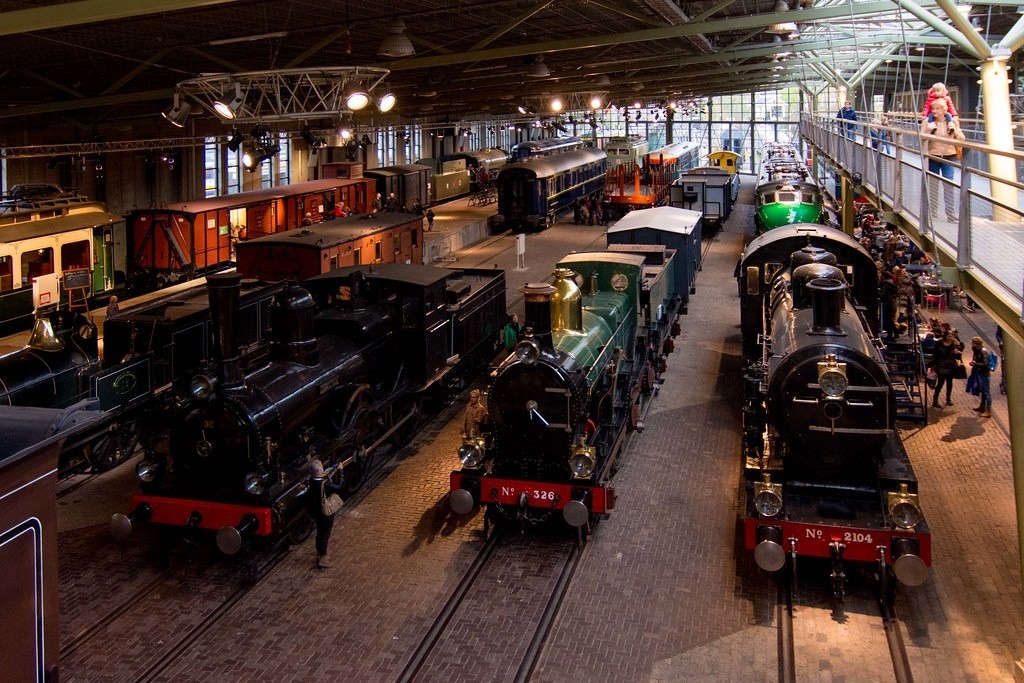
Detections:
[318,554,333,570]
[315,553,330,565]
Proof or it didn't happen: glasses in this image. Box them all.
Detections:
[469,395,478,399]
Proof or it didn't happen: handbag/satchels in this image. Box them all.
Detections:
[320,479,344,517]
[926,367,937,391]
[948,356,967,379]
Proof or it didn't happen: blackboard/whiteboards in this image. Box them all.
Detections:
[62,267,91,291]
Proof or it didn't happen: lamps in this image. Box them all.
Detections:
[301,124,320,148]
[766,37,791,83]
[161,84,191,128]
[259,144,280,160]
[346,81,370,111]
[766,2,797,32]
[228,128,243,152]
[376,12,415,58]
[371,81,397,114]
[242,146,265,172]
[212,82,246,119]
[341,129,353,139]
[525,55,550,77]
[551,58,582,78]
[595,74,611,85]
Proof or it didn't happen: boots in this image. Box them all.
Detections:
[972,403,985,413]
[933,394,942,408]
[945,393,953,405]
[979,406,991,417]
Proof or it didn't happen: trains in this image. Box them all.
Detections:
[0,137,931,598]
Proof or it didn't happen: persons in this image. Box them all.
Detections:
[881,117,893,155]
[854,201,965,408]
[230,223,246,252]
[309,459,344,568]
[459,389,487,438]
[504,314,522,357]
[107,296,119,316]
[480,169,488,187]
[969,336,992,417]
[922,82,959,135]
[302,192,435,233]
[919,99,965,224]
[573,189,613,226]
[869,120,882,149]
[837,101,858,139]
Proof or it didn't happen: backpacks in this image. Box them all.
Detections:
[979,347,998,372]
